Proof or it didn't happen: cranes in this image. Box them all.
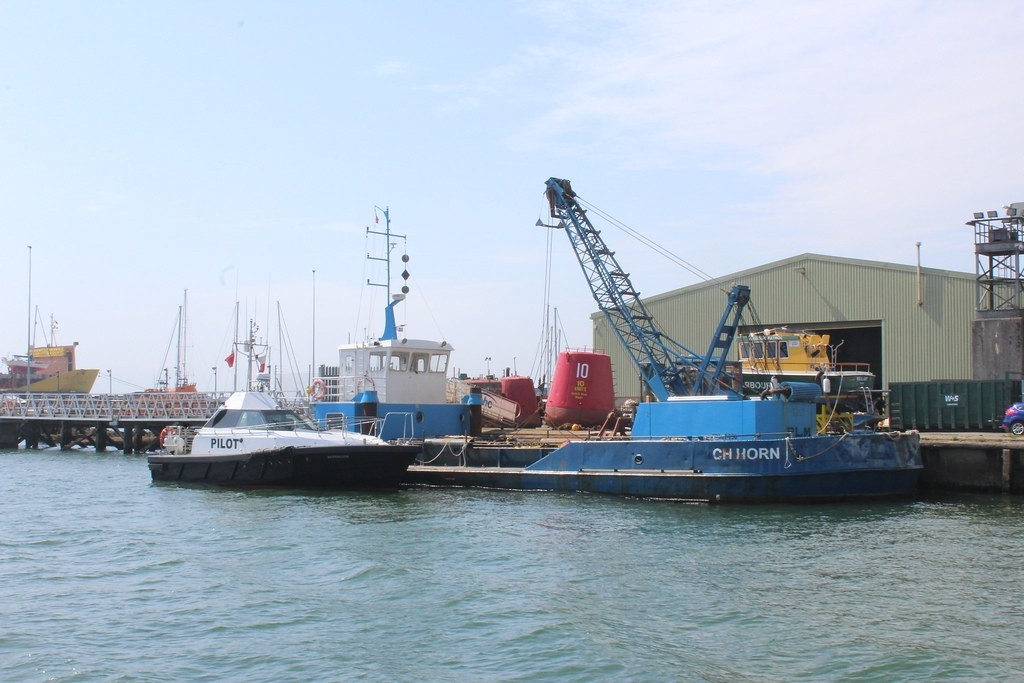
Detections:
[536,178,891,429]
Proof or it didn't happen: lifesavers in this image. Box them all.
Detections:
[161,427,175,447]
[311,380,326,399]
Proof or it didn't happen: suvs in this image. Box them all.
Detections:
[1002,404,1024,435]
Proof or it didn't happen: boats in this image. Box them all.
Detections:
[43,426,154,450]
[145,392,421,492]
[402,382,922,500]
[133,289,224,415]
[0,245,100,393]
